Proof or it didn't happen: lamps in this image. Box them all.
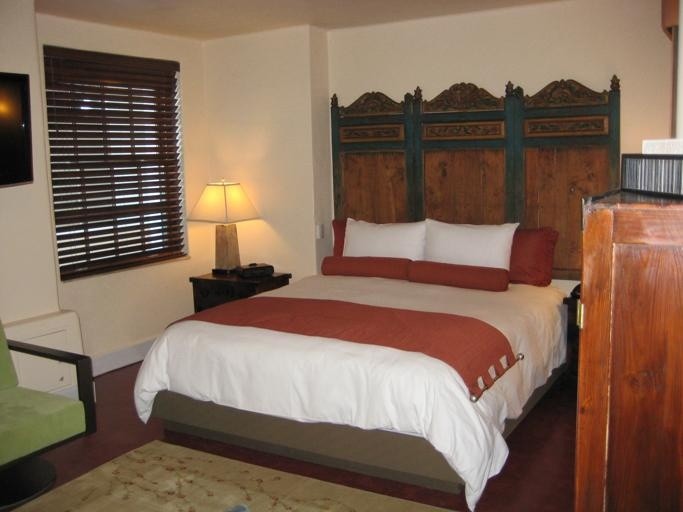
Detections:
[183,182,263,272]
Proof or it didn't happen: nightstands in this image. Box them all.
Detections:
[188,267,291,316]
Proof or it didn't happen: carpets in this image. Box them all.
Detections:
[3,435,459,512]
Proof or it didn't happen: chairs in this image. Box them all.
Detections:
[0,321,98,511]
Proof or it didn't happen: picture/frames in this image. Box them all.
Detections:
[0,71,35,185]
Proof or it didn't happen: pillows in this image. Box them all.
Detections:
[318,214,560,291]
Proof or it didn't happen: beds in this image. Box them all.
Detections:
[147,260,577,498]
